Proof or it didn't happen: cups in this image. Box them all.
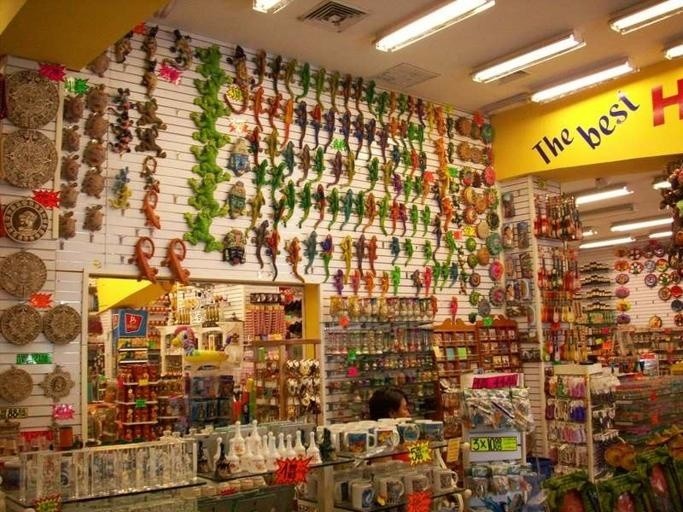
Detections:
[315,418,464,512]
[298,473,319,501]
[61,457,83,488]
[0,456,21,490]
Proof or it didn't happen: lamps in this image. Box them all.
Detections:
[663,40,683,60]
[372,0,496,52]
[577,237,636,249]
[607,0,683,37]
[252,0,292,14]
[651,180,672,190]
[470,30,587,86]
[529,58,639,105]
[648,230,673,239]
[609,216,676,232]
[582,226,598,237]
[575,183,634,205]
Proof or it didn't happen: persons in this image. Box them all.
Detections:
[369,389,409,420]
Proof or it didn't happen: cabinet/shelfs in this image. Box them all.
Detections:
[0,258,683,512]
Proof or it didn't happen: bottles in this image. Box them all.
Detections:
[197,419,337,479]
[579,260,613,311]
[117,364,158,441]
[329,295,434,323]
